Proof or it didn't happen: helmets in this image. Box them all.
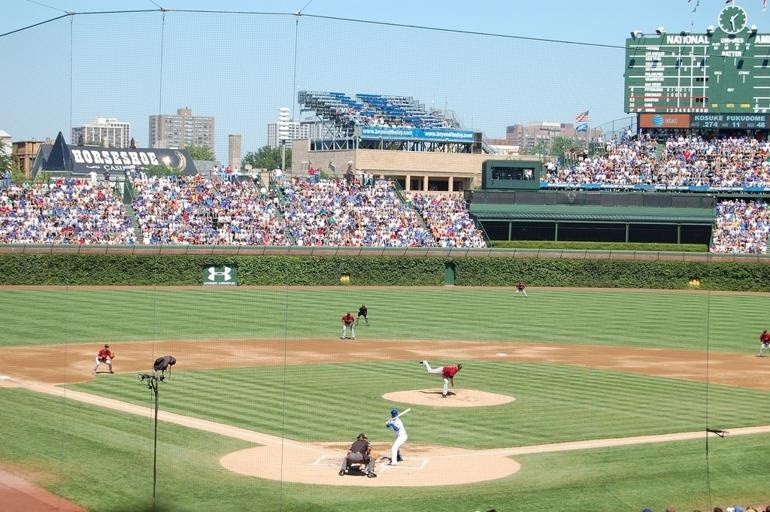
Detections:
[458,364,462,369]
[357,434,366,440]
[391,410,397,417]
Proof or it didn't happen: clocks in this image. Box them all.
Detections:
[718,5,747,34]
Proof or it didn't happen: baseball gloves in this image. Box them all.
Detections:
[371,452,380,460]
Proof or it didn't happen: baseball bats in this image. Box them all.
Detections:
[386,408,411,425]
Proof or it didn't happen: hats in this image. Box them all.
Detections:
[643,509,651,512]
[727,506,742,512]
[667,506,674,511]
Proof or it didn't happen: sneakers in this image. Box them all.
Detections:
[367,473,376,477]
[339,471,344,476]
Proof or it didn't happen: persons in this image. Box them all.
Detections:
[539,126,770,254]
[420,360,462,398]
[136,165,291,248]
[339,433,377,477]
[269,161,486,247]
[1,169,140,245]
[92,344,114,374]
[756,329,770,356]
[387,409,408,466]
[341,312,355,339]
[355,304,369,325]
[513,282,527,297]
[644,505,770,512]
[336,106,454,128]
[387,101,392,106]
